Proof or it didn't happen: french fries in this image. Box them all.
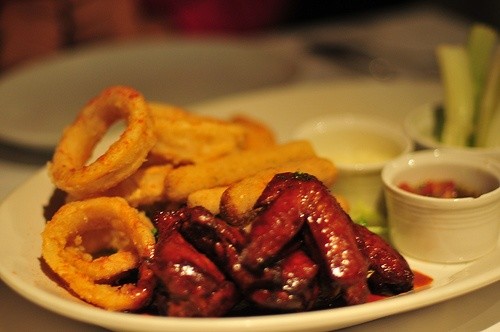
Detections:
[63,103,349,222]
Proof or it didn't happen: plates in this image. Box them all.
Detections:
[0,40,301,150]
[0,86,500,332]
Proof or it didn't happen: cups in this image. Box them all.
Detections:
[406,99,500,162]
[294,119,414,234]
[381,150,500,265]
